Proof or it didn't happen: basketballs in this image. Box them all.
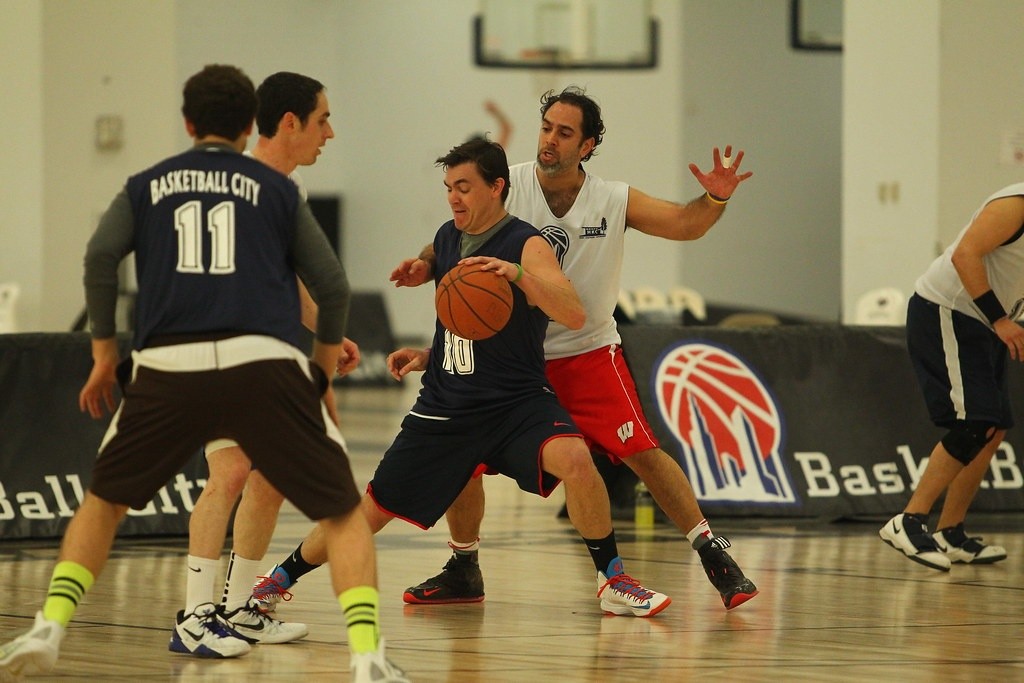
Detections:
[434,263,514,341]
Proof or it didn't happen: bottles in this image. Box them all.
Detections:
[635,479,654,528]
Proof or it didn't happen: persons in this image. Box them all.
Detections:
[255,139,670,617]
[171,72,360,658]
[878,179,1024,571]
[0,66,412,683]
[389,90,758,611]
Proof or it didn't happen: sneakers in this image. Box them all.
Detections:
[349,636,411,683]
[402,553,485,604]
[169,602,251,658]
[251,563,298,612]
[0,611,66,679]
[879,512,951,571]
[697,536,760,610]
[932,521,1007,565]
[216,594,309,644]
[596,555,672,618]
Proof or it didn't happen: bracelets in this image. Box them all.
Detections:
[515,263,522,280]
[973,289,1007,324]
[707,191,727,205]
